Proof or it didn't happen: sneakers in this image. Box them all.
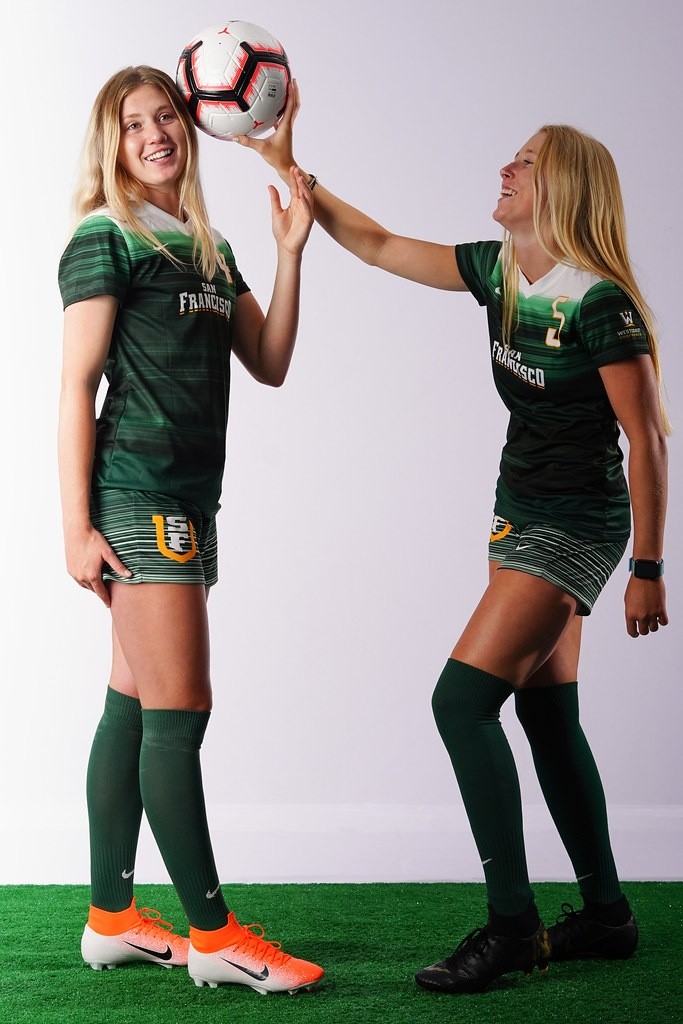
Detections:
[81,897,190,970]
[188,911,324,995]
[547,909,638,962]
[415,918,551,994]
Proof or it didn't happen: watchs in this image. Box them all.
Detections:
[626,558,665,579]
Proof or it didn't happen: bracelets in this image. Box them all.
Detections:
[306,173,318,190]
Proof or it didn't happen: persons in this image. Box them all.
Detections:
[234,79,668,996]
[59,62,325,996]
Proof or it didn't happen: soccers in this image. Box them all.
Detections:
[175,20,291,141]
